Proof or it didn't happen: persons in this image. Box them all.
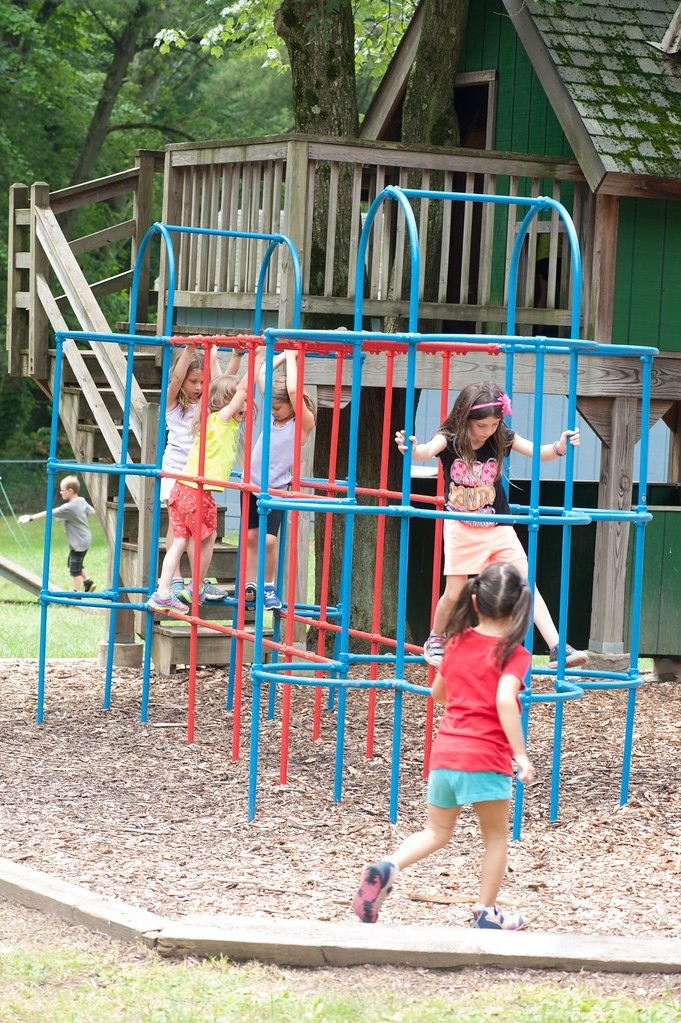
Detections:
[18,476,97,600]
[239,349,317,611]
[352,561,535,932]
[395,381,588,668]
[147,334,267,615]
[160,334,227,601]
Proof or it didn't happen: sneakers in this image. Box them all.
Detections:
[244,582,257,611]
[545,642,589,669]
[147,592,190,616]
[181,582,206,604]
[190,579,228,601]
[170,577,185,602]
[424,631,446,668]
[468,904,524,931]
[352,860,395,923]
[264,584,282,611]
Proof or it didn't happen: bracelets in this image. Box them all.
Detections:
[554,441,567,456]
[233,349,246,356]
[30,515,33,521]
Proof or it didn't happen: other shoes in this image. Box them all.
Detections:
[66,590,82,600]
[83,578,97,592]
